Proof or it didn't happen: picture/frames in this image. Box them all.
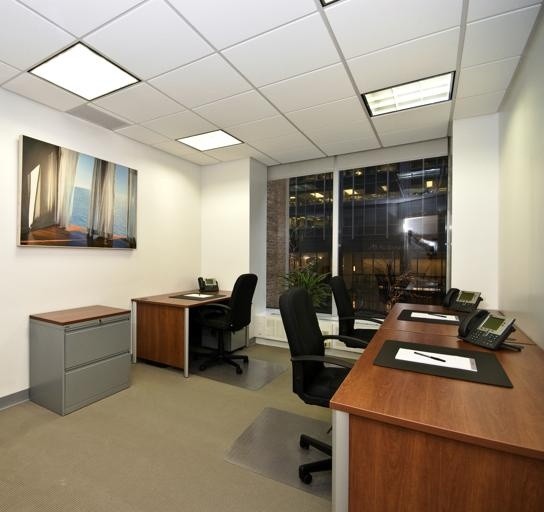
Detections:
[16,134,139,251]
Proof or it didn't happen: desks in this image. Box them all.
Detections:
[328,328,543,511]
[380,302,536,345]
[131,289,249,378]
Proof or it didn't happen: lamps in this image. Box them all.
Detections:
[361,70,456,117]
[175,129,245,152]
[27,41,141,101]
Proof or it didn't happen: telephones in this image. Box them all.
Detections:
[443,287,483,313]
[198,277,219,292]
[457,308,516,351]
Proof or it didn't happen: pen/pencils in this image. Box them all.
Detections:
[429,313,447,318]
[416,352,446,363]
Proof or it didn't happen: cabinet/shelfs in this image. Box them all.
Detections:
[29,305,131,417]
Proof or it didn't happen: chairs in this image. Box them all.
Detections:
[279,287,369,484]
[329,276,388,349]
[199,273,258,375]
